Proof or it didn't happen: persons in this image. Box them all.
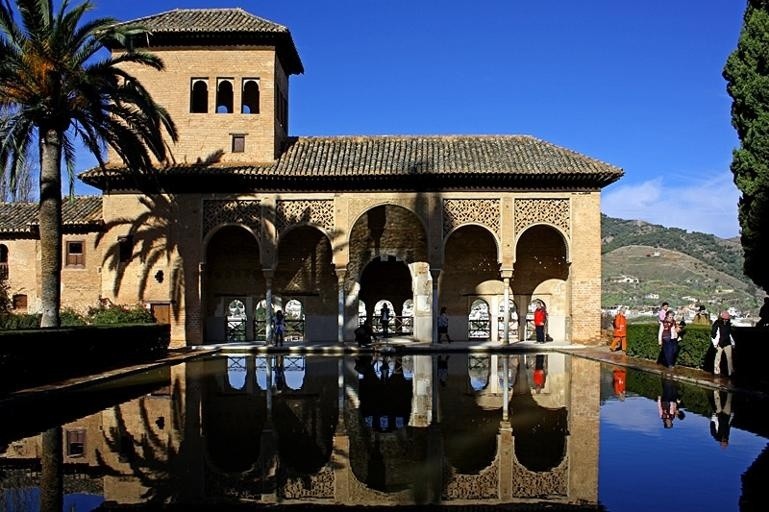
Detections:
[610,305,628,355]
[534,354,545,394]
[709,310,738,378]
[379,356,389,386]
[533,302,546,344]
[379,303,390,336]
[710,389,734,450]
[613,364,627,402]
[657,310,679,368]
[437,354,450,390]
[437,307,454,344]
[671,376,684,420]
[660,302,670,323]
[274,356,286,390]
[656,378,677,430]
[272,310,286,347]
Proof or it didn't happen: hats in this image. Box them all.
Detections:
[720,311,730,320]
